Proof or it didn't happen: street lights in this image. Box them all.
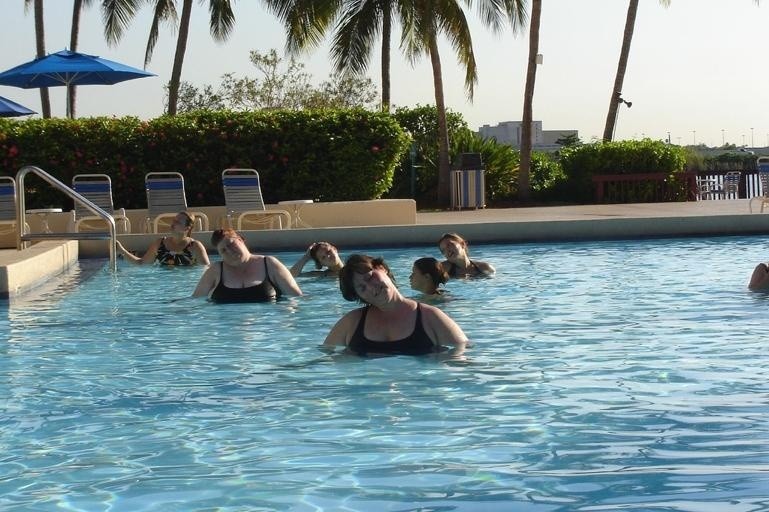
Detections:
[639,126,769,149]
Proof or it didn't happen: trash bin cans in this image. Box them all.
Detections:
[450,153,487,210]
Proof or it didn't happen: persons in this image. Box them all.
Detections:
[436,231,497,283]
[748,262,769,290]
[113,209,211,268]
[321,253,471,361]
[288,241,346,280]
[169,225,306,305]
[405,257,458,306]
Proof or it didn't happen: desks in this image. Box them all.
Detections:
[26,209,62,232]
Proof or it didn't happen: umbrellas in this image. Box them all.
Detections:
[0,95,39,123]
[0,46,160,121]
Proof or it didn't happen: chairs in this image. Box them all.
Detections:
[69,173,132,233]
[144,171,210,233]
[221,168,291,230]
[700,171,742,200]
[749,156,769,213]
[0,176,30,249]
